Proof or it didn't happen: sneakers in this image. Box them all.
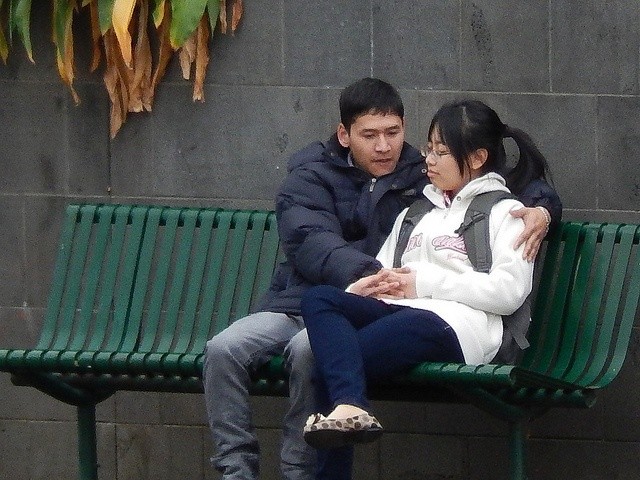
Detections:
[303,412,382,449]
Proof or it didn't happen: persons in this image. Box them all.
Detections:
[202,75,562,480]
[299,97,536,451]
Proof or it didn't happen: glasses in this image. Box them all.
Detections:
[420,141,478,158]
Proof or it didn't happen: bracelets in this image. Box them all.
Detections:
[537,207,551,237]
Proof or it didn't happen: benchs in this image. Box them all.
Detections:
[1,201,640,480]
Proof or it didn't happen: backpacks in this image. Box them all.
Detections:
[394,191,547,364]
